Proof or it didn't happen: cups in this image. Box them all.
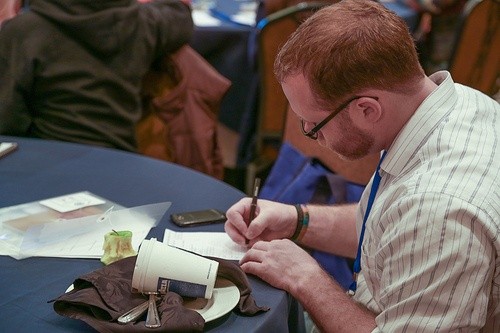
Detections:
[131,237,219,299]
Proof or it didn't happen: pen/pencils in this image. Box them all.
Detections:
[244,178,261,246]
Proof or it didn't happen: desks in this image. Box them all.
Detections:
[191,1,418,55]
[0,135,306,333]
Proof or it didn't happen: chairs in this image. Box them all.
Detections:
[235,0,335,195]
[445,0,500,99]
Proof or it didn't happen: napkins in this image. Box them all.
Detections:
[47,255,272,333]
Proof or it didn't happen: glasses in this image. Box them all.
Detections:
[300,95,379,140]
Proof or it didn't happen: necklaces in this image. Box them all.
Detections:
[349,150,388,295]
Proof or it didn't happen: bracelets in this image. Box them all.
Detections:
[290,204,304,242]
[295,204,310,241]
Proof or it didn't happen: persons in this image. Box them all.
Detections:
[224,0,500,333]
[1,0,194,150]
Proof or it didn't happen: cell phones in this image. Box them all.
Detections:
[0,141,18,158]
[170,208,227,227]
